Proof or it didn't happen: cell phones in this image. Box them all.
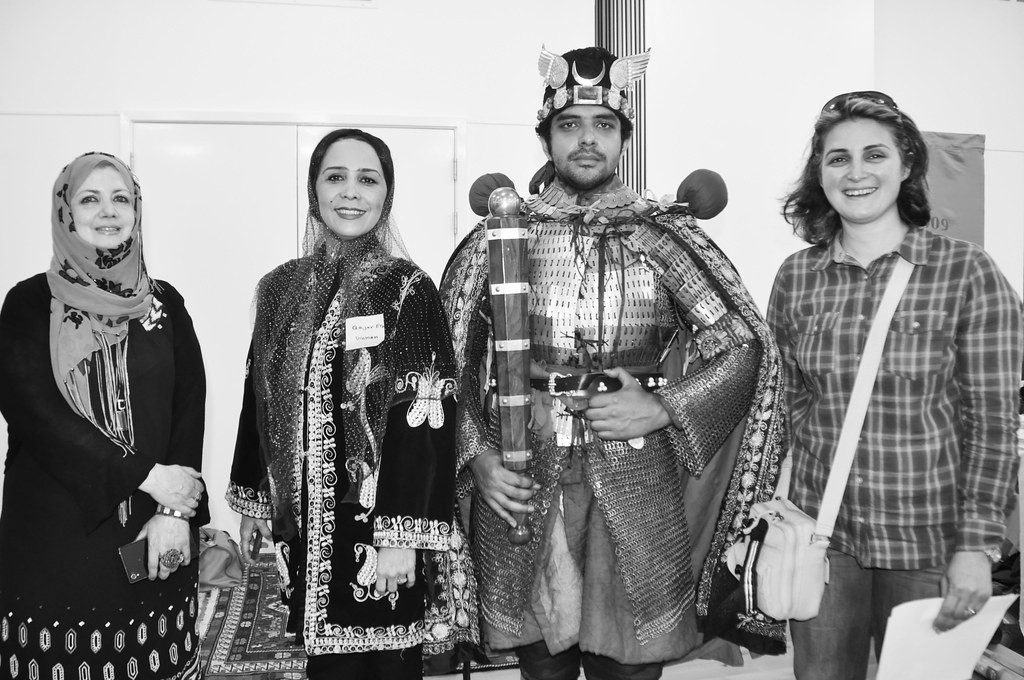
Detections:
[119,532,199,584]
[251,531,263,560]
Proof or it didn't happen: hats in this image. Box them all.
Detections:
[537,45,651,144]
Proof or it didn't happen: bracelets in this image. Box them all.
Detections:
[156,502,190,522]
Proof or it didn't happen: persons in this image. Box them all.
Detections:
[0,150,212,680]
[225,45,782,680]
[764,90,1024,680]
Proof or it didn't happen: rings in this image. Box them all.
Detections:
[162,548,183,569]
[396,578,408,583]
[967,607,976,615]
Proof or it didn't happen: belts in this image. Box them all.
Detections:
[529,369,665,396]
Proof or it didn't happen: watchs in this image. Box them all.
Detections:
[983,546,1001,563]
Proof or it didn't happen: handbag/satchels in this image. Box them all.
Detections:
[194,550,310,680]
[724,496,831,622]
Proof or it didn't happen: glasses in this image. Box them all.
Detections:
[821,90,897,113]
